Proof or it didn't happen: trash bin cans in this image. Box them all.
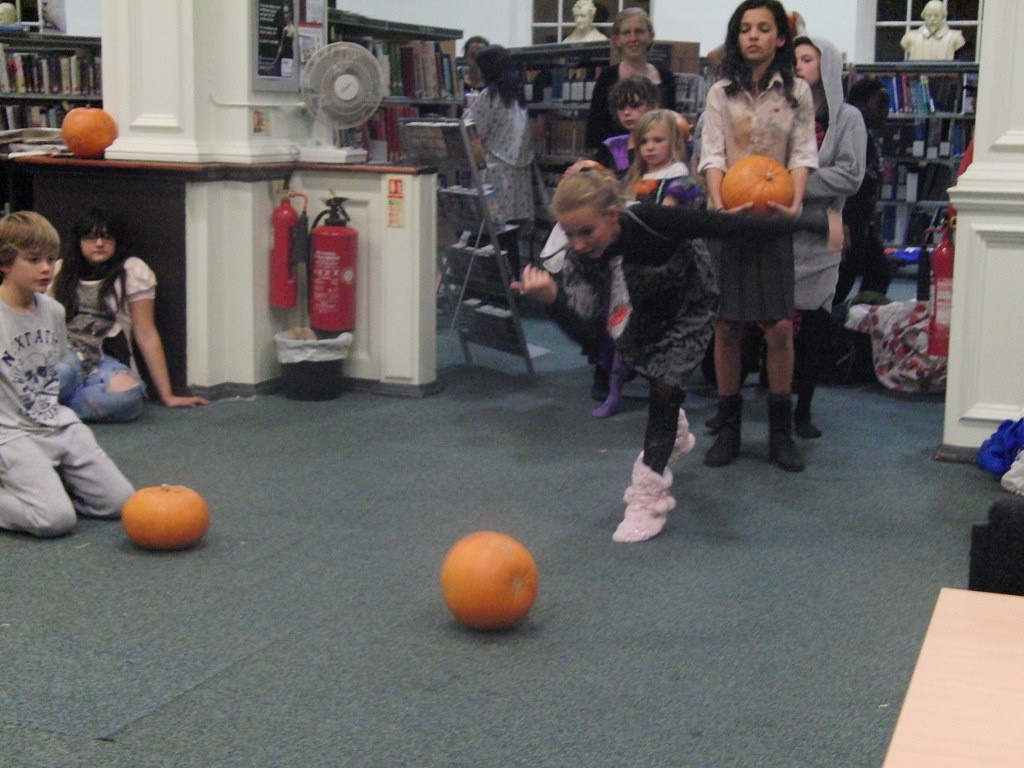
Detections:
[274,332,352,402]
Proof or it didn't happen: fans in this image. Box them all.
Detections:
[301,43,384,128]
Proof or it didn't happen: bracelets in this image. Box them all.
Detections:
[713,206,725,211]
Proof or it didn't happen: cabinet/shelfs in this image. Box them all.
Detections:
[851,61,980,247]
[509,42,700,280]
[0,29,102,131]
[330,7,464,160]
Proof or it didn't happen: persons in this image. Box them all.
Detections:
[508,162,844,544]
[833,75,894,310]
[44,207,212,424]
[559,0,609,42]
[901,0,967,61]
[0,210,137,535]
[700,35,862,438]
[576,6,695,416]
[461,35,535,286]
[694,1,819,472]
[269,5,296,69]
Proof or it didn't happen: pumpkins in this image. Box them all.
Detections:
[442,531,536,630]
[124,483,209,547]
[564,160,606,176]
[721,154,793,215]
[63,104,116,157]
[666,110,690,137]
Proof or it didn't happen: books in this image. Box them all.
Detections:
[548,116,587,158]
[323,25,455,166]
[0,43,101,159]
[858,73,977,245]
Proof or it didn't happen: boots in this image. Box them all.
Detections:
[612,450,677,543]
[703,393,741,467]
[664,406,695,459]
[793,372,823,438]
[705,412,721,431]
[766,394,806,472]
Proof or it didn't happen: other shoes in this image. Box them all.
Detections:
[590,353,612,402]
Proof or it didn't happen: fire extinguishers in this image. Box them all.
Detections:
[308,199,367,330]
[927,215,958,357]
[269,189,308,309]
